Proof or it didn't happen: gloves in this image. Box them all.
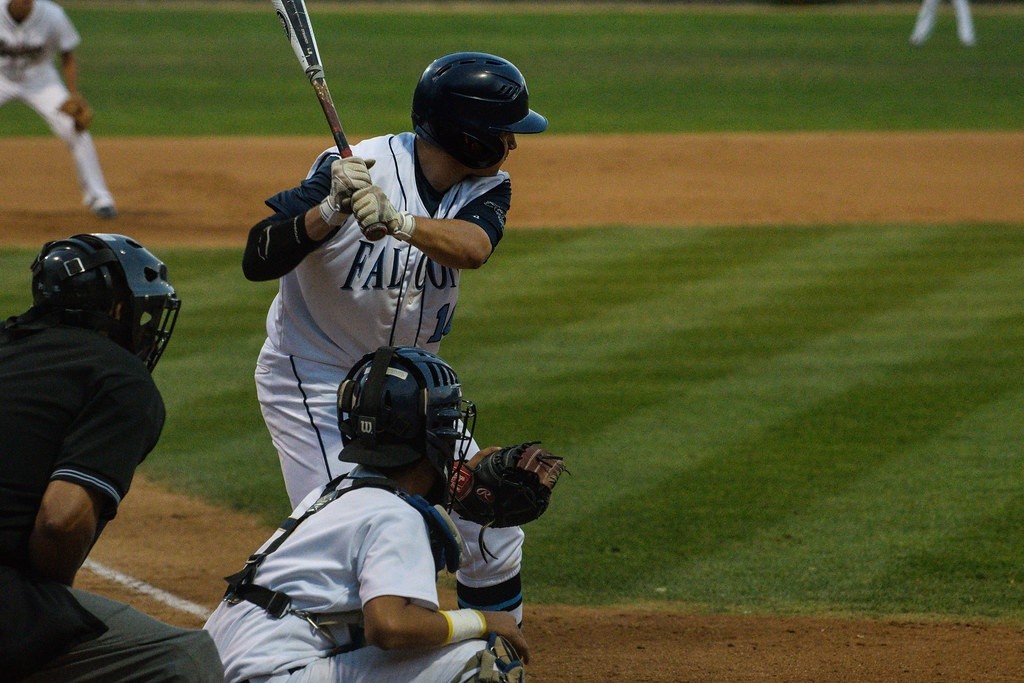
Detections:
[319,157,376,225]
[342,185,416,241]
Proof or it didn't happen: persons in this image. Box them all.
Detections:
[0,232,225,683]
[202,346,571,683]
[241,52,550,639]
[0,0,118,219]
[908,0,978,46]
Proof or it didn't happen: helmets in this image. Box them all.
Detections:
[336,345,477,515]
[30,233,182,374]
[411,52,547,170]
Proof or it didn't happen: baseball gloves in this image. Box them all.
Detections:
[446,439,567,531]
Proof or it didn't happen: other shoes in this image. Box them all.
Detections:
[96,207,118,218]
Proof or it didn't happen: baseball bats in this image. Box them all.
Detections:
[270,0,389,242]
